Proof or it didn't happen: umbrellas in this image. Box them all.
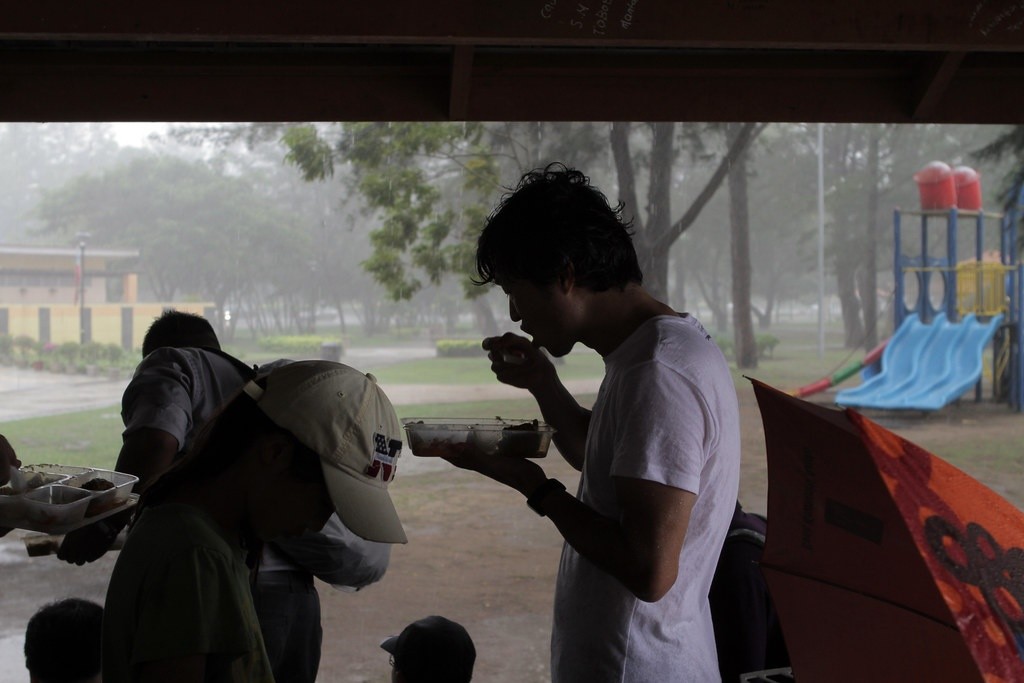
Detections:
[743,375,1024,683]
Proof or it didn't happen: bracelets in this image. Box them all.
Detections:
[527,478,566,517]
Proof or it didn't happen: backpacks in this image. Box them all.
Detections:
[708,511,792,683]
[192,347,395,588]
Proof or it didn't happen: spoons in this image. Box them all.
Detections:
[495,344,528,366]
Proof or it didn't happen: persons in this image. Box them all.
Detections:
[101,360,409,683]
[440,163,740,683]
[707,500,795,683]
[23,598,104,683]
[56,311,324,683]
[378,616,477,683]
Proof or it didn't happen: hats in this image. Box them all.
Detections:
[242,360,409,545]
[379,616,476,683]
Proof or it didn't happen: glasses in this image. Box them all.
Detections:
[389,654,396,668]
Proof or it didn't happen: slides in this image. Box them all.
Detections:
[834,311,1005,412]
